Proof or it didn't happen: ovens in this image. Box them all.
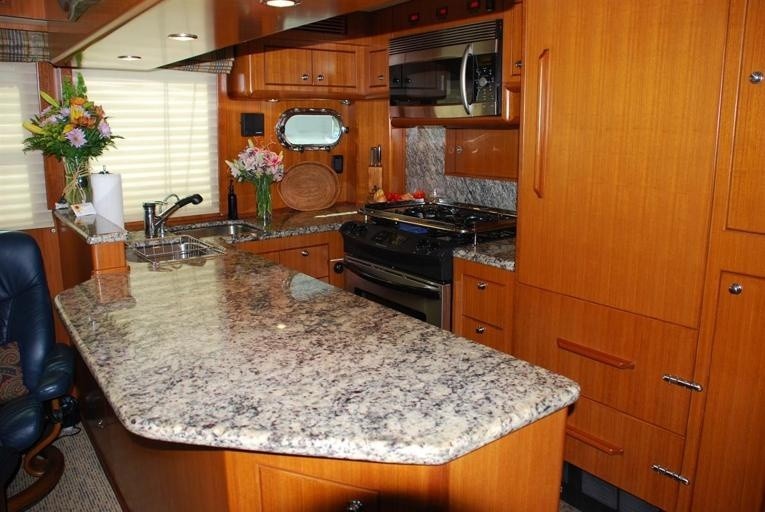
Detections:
[343,254,451,333]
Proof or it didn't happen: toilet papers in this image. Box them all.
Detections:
[91,172,126,238]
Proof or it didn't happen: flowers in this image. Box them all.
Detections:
[20,71,124,204]
[224,137,286,216]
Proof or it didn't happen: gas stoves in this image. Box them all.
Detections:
[339,201,516,285]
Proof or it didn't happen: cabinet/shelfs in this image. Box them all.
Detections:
[447,2,522,182]
[227,39,366,100]
[79,365,228,511]
[227,451,450,512]
[367,44,389,100]
[232,233,344,283]
[388,63,450,101]
[518,0,731,330]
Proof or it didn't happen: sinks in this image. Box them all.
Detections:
[157,219,265,240]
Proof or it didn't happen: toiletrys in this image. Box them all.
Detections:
[227,178,239,220]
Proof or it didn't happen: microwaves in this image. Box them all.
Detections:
[388,18,502,118]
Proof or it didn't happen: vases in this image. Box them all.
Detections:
[255,179,273,236]
[64,175,91,216]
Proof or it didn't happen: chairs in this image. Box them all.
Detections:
[0,231,78,511]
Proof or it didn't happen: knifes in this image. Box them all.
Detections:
[370,144,381,167]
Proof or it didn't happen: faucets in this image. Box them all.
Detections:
[158,193,179,215]
[142,193,204,238]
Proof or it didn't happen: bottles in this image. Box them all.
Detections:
[228,180,236,219]
